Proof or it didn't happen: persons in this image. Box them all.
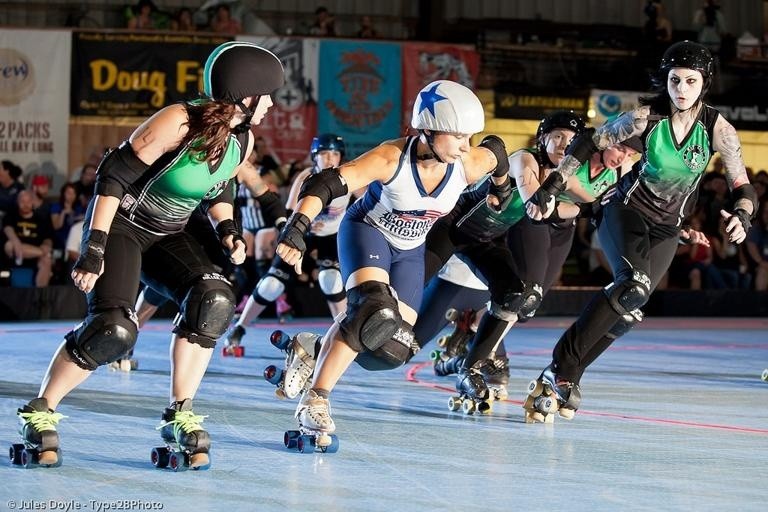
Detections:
[521,36,759,427]
[209,4,244,34]
[644,1,673,45]
[170,6,199,32]
[4,107,766,405]
[308,6,344,37]
[276,75,509,433]
[127,0,160,36]
[6,39,289,454]
[687,1,730,54]
[356,15,383,38]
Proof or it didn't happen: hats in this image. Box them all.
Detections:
[32,175,49,186]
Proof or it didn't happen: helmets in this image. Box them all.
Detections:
[661,41,714,80]
[203,41,284,104]
[412,79,485,135]
[310,133,345,161]
[604,112,648,153]
[536,111,585,140]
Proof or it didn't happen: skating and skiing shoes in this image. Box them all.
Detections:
[523,365,582,424]
[151,399,210,471]
[223,326,246,357]
[108,348,139,372]
[264,330,323,400]
[284,387,338,453]
[275,294,292,323]
[9,395,63,468]
[430,307,508,414]
[233,295,251,319]
